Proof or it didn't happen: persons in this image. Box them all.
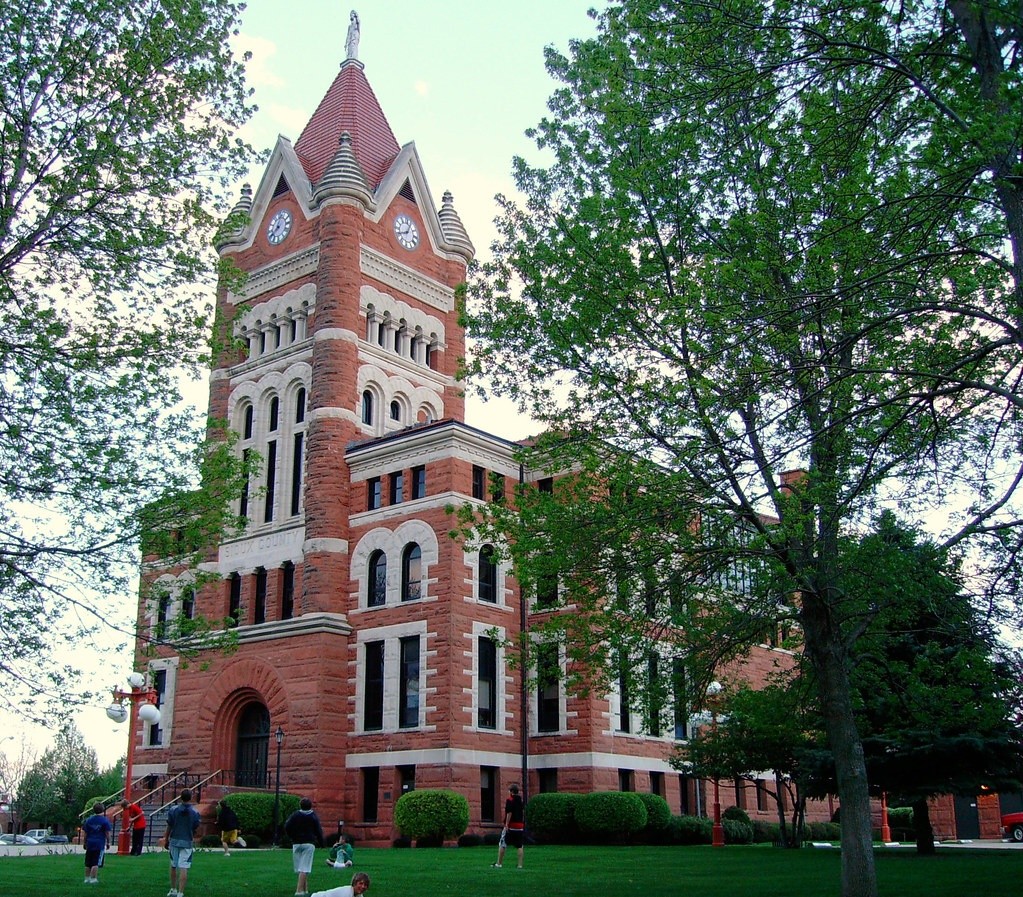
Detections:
[120,799,146,857]
[489,784,525,869]
[326,834,354,868]
[82,803,112,884]
[309,872,370,897]
[285,797,323,895]
[164,788,200,897]
[214,800,246,857]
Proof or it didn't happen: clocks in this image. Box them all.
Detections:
[393,214,420,250]
[268,209,291,245]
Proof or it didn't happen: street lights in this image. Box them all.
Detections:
[689,681,740,845]
[272,723,285,846]
[105,672,162,856]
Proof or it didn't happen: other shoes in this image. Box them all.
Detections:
[167,888,178,896]
[84,878,99,884]
[490,863,502,869]
[295,890,309,896]
[177,892,185,897]
[224,853,230,856]
[238,837,247,847]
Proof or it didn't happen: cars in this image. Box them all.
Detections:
[999,812,1023,842]
[1,828,69,846]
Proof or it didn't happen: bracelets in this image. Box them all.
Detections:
[107,842,110,843]
